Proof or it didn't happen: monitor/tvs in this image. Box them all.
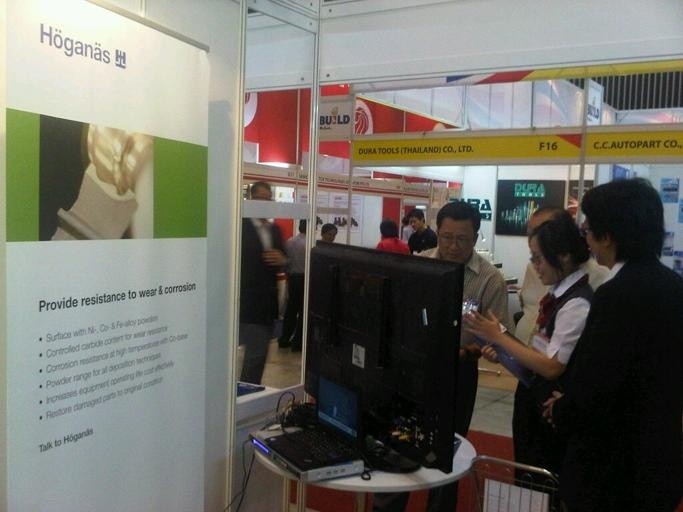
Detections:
[305,240,466,474]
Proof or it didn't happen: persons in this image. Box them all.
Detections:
[275,207,438,353]
[239,180,292,385]
[462,218,596,494]
[507,204,613,492]
[370,200,513,511]
[540,176,682,510]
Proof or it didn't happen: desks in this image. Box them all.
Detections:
[250,424,476,512]
